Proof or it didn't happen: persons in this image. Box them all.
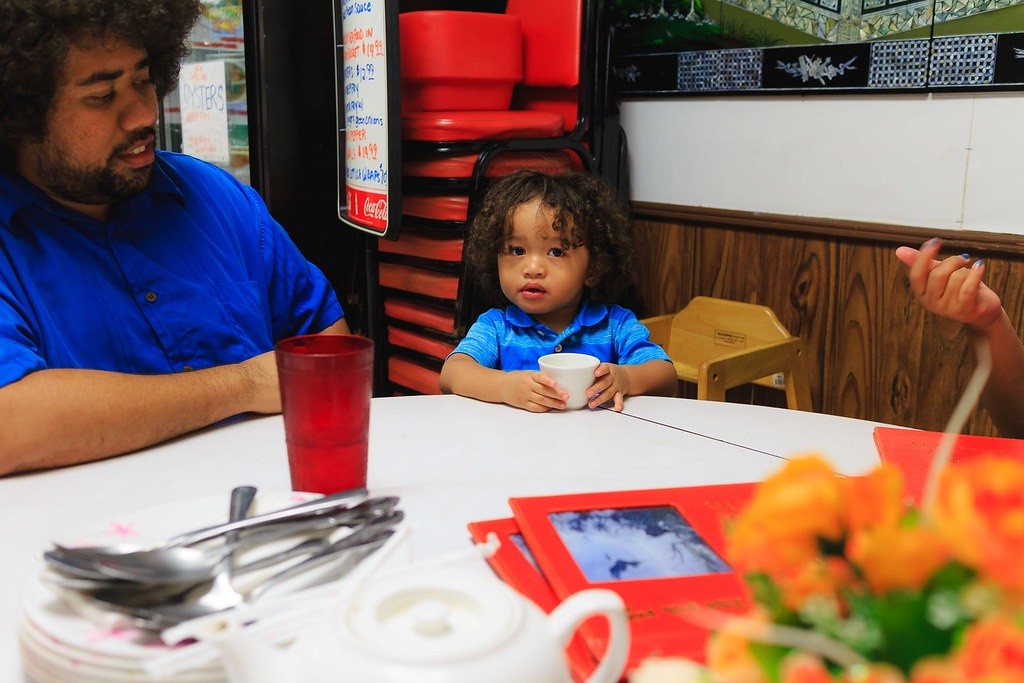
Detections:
[0,0,349,475]
[437,171,678,413]
[896,237,1024,440]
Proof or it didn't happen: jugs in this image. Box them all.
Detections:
[353,572,629,682]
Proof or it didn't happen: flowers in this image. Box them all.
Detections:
[702,457,1024,683]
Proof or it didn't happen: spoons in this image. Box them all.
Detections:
[45,486,405,633]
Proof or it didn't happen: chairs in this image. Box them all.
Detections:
[366,1,628,400]
[639,296,812,413]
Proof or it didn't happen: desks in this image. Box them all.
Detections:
[1,396,1024,683]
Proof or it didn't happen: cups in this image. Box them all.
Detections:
[275,333,374,493]
[538,353,601,409]
